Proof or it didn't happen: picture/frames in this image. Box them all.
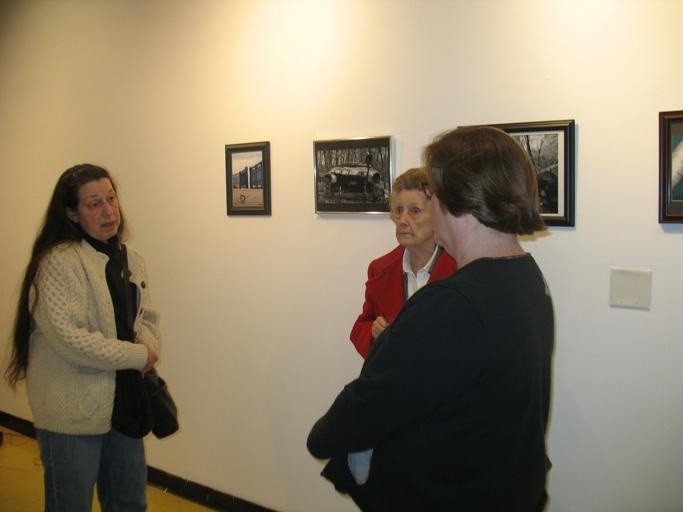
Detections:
[313,137,391,214]
[482,120,575,227]
[225,141,271,215]
[658,111,683,224]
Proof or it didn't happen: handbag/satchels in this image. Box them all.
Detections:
[120,241,179,439]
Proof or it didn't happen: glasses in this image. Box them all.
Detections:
[421,181,438,199]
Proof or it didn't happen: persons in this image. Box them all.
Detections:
[306,125,555,512]
[350,169,457,362]
[5,164,162,512]
